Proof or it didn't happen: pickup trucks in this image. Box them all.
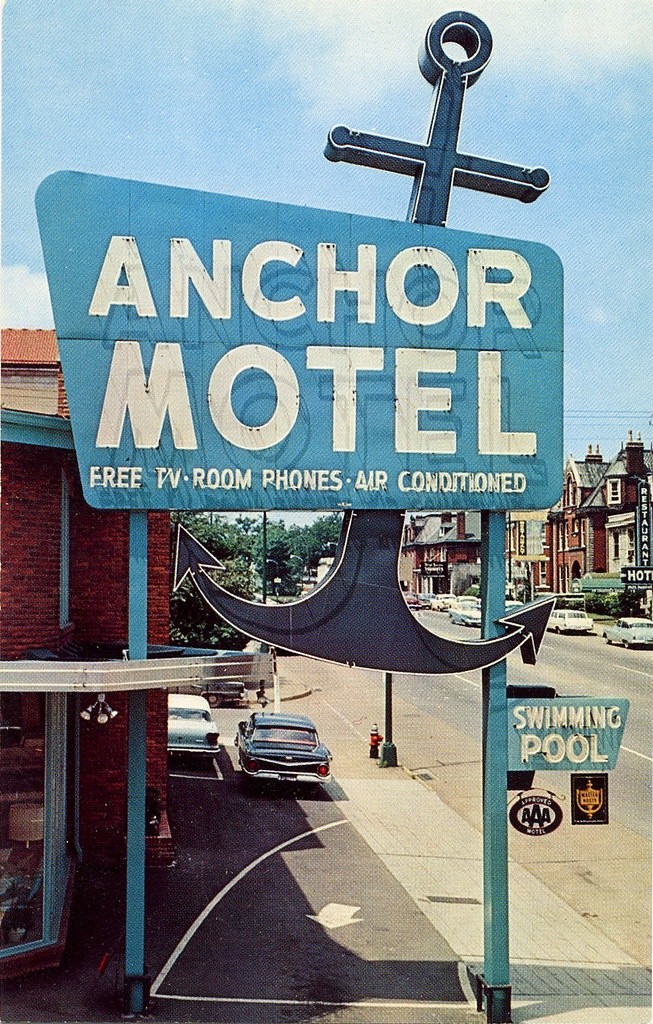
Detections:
[546,609,595,635]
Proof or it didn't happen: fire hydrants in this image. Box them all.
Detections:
[369,721,384,758]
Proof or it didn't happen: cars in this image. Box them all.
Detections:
[166,692,221,765]
[504,600,524,616]
[168,682,244,708]
[305,566,317,576]
[402,591,482,627]
[235,711,333,785]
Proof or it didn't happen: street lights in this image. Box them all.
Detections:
[327,541,339,547]
[265,558,279,606]
[290,554,304,598]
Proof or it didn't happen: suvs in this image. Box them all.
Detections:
[602,617,653,648]
